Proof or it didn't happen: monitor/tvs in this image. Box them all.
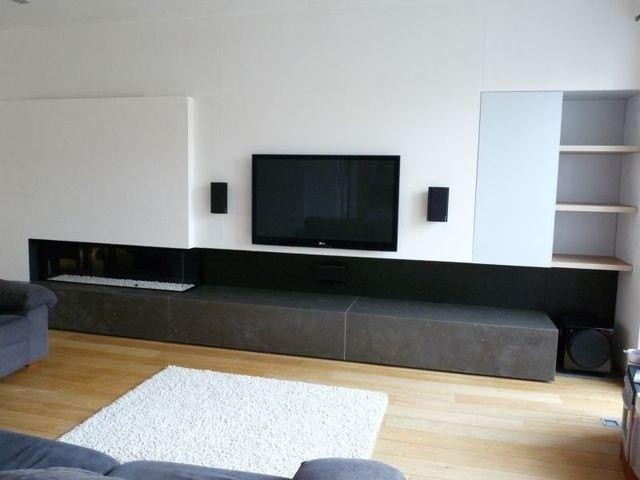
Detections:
[251,153,401,252]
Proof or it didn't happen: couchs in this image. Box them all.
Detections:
[0,423,404,480]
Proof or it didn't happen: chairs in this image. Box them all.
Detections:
[0,279,58,378]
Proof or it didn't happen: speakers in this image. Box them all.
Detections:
[210,182,229,215]
[562,326,615,373]
[426,186,450,222]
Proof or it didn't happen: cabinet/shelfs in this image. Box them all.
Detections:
[477,90,639,272]
[39,274,559,381]
[619,365,640,479]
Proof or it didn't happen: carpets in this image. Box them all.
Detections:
[56,365,391,477]
